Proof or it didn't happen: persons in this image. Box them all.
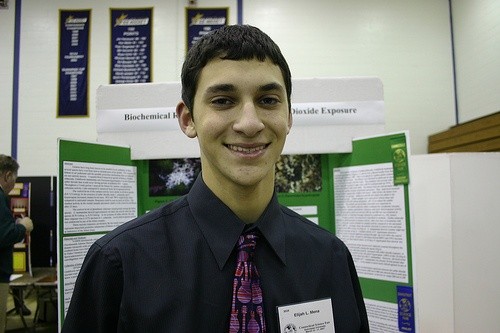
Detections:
[0,154,33,333]
[60,24,370,333]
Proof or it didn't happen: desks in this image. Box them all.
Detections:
[5,274,58,333]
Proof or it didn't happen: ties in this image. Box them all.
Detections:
[230,235,266,333]
